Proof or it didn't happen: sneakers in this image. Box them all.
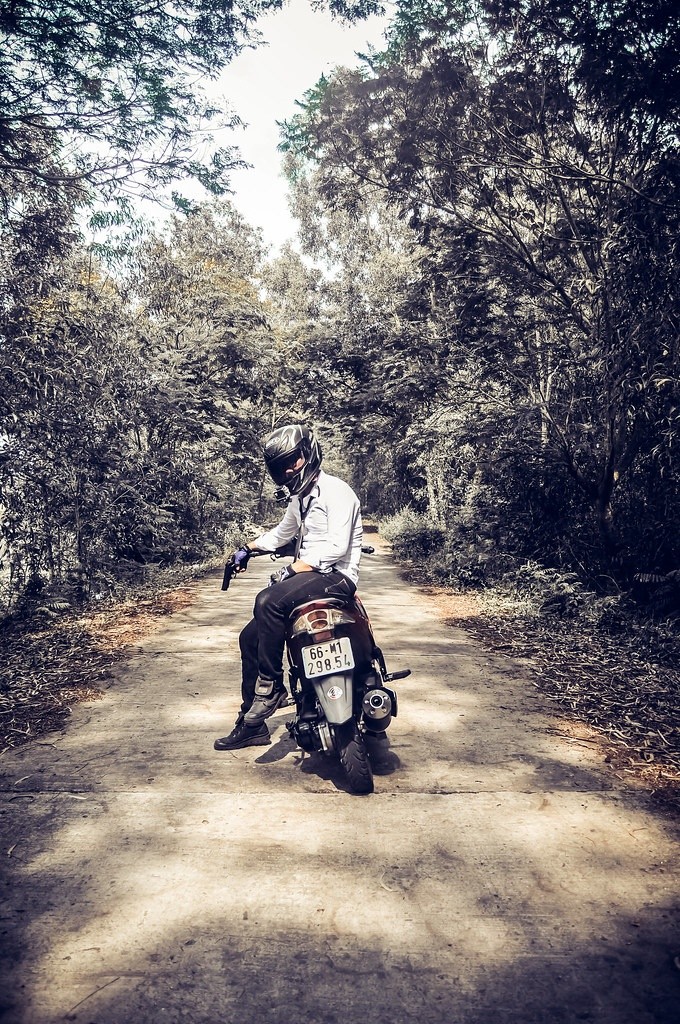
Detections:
[244,676,288,727]
[214,711,272,750]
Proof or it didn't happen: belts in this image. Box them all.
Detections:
[332,568,357,591]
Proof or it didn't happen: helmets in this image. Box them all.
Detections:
[263,425,323,503]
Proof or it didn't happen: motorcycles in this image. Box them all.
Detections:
[233,544,413,794]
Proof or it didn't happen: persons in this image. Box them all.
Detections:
[212,424,363,750]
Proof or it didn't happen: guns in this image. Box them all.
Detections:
[220,554,250,592]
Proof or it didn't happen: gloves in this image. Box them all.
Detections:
[267,563,297,587]
[226,543,252,578]
